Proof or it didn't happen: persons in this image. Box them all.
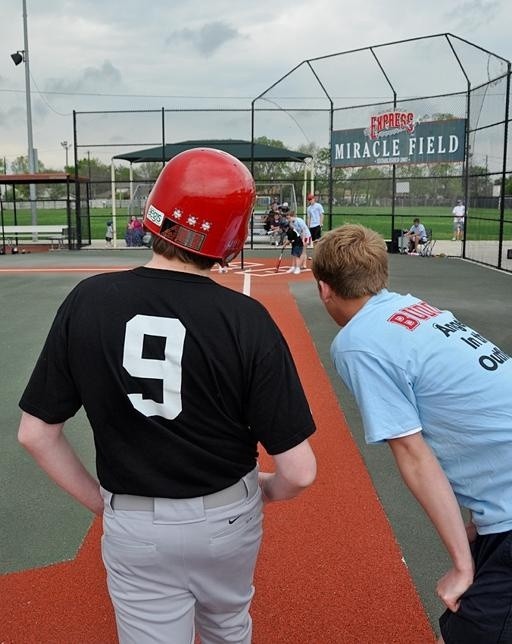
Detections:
[18,147,318,644]
[264,194,325,275]
[105,219,114,248]
[403,218,427,253]
[452,200,465,241]
[312,223,512,644]
[126,216,143,246]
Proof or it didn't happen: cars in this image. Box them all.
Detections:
[331,193,453,206]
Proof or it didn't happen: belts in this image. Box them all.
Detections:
[96,464,260,514]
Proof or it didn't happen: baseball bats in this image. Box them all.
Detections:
[275,247,285,273]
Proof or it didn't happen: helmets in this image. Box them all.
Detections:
[142,146,258,267]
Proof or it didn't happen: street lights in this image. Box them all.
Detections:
[61,141,72,167]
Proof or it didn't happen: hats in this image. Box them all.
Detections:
[306,195,315,201]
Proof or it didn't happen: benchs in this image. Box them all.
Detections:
[47,227,76,251]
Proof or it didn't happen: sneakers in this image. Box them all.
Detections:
[286,264,307,275]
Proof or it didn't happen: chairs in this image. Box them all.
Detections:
[410,227,431,256]
